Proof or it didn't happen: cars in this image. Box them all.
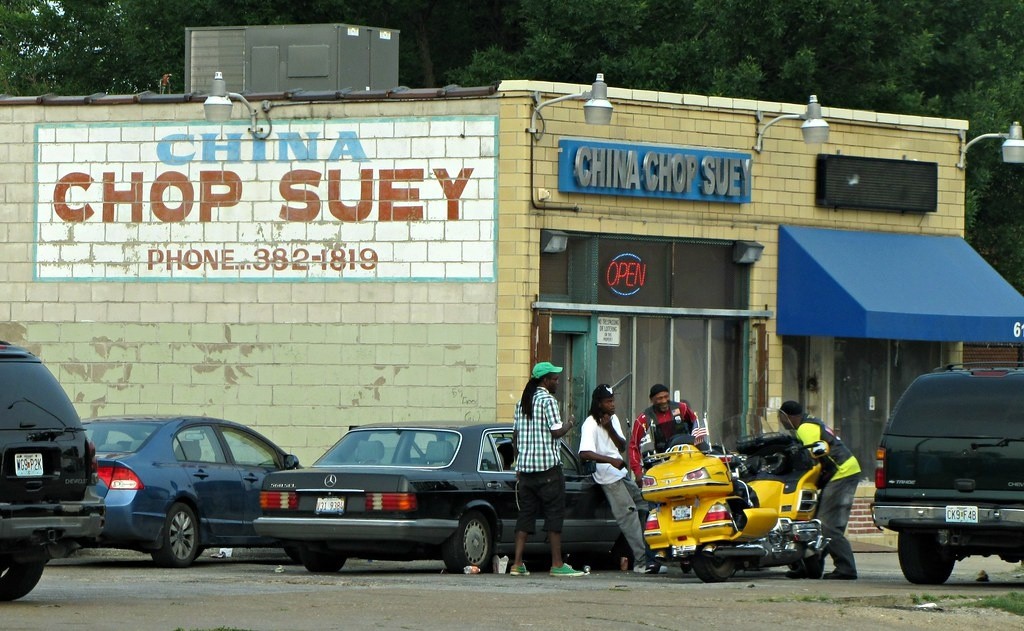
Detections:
[82,414,303,569]
[252,420,635,574]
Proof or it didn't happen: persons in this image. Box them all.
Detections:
[578,384,667,574]
[509,362,584,576]
[778,401,862,580]
[628,384,707,573]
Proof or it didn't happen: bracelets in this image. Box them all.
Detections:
[569,421,574,426]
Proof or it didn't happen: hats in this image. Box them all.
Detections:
[779,400,802,415]
[531,362,564,378]
[649,383,669,399]
[593,383,620,399]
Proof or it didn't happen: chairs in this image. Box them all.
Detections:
[666,434,759,479]
[347,439,504,472]
[98,440,202,461]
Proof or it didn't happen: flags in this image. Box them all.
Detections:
[691,416,710,446]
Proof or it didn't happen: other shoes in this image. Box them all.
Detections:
[822,569,858,580]
[786,566,822,580]
[633,564,667,574]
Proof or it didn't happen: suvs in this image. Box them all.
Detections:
[870,362,1024,584]
[0,340,99,604]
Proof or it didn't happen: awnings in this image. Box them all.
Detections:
[776,224,1024,343]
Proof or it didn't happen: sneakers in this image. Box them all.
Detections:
[550,563,584,577]
[510,563,530,576]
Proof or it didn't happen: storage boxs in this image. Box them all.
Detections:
[642,444,734,503]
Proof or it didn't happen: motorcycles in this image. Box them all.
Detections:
[641,406,833,583]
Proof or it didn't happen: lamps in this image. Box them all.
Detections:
[733,241,764,264]
[541,230,569,252]
[955,121,1024,169]
[203,72,259,127]
[525,73,614,133]
[752,95,829,152]
